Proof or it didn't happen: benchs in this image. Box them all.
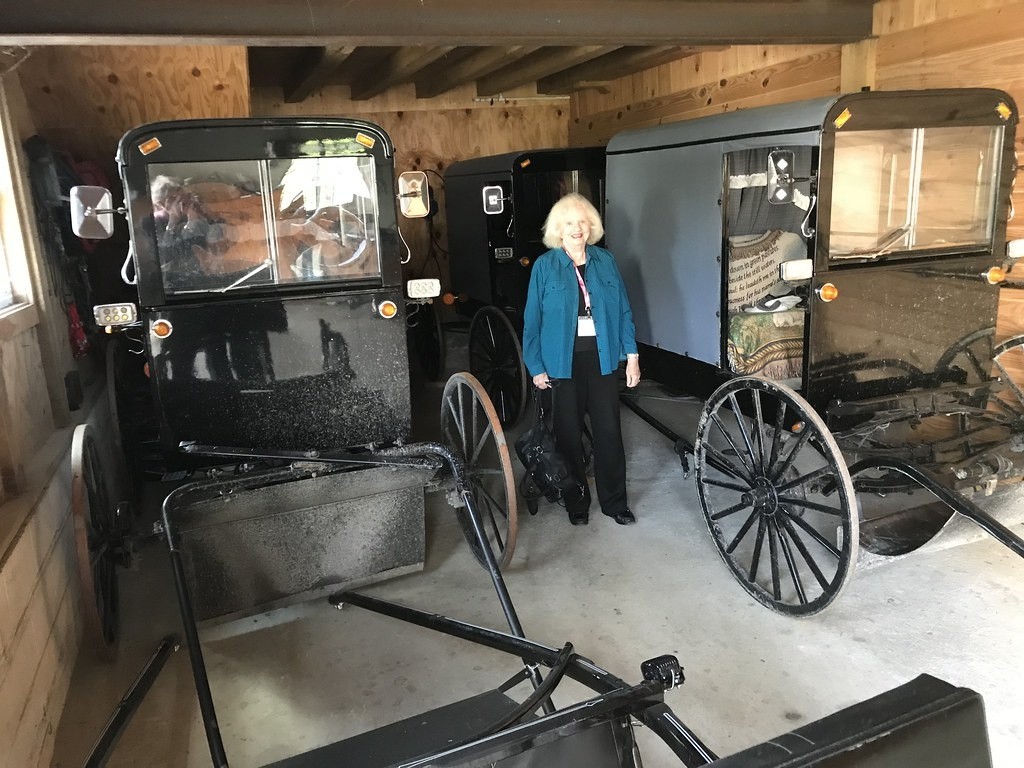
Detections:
[726,231,810,389]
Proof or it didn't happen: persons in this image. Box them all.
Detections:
[522,191,641,526]
[146,175,230,291]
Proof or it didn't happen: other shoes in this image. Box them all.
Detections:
[568,508,590,526]
[601,506,636,525]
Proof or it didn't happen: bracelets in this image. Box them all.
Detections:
[165,225,175,236]
[628,354,639,358]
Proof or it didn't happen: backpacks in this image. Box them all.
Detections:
[513,382,592,516]
